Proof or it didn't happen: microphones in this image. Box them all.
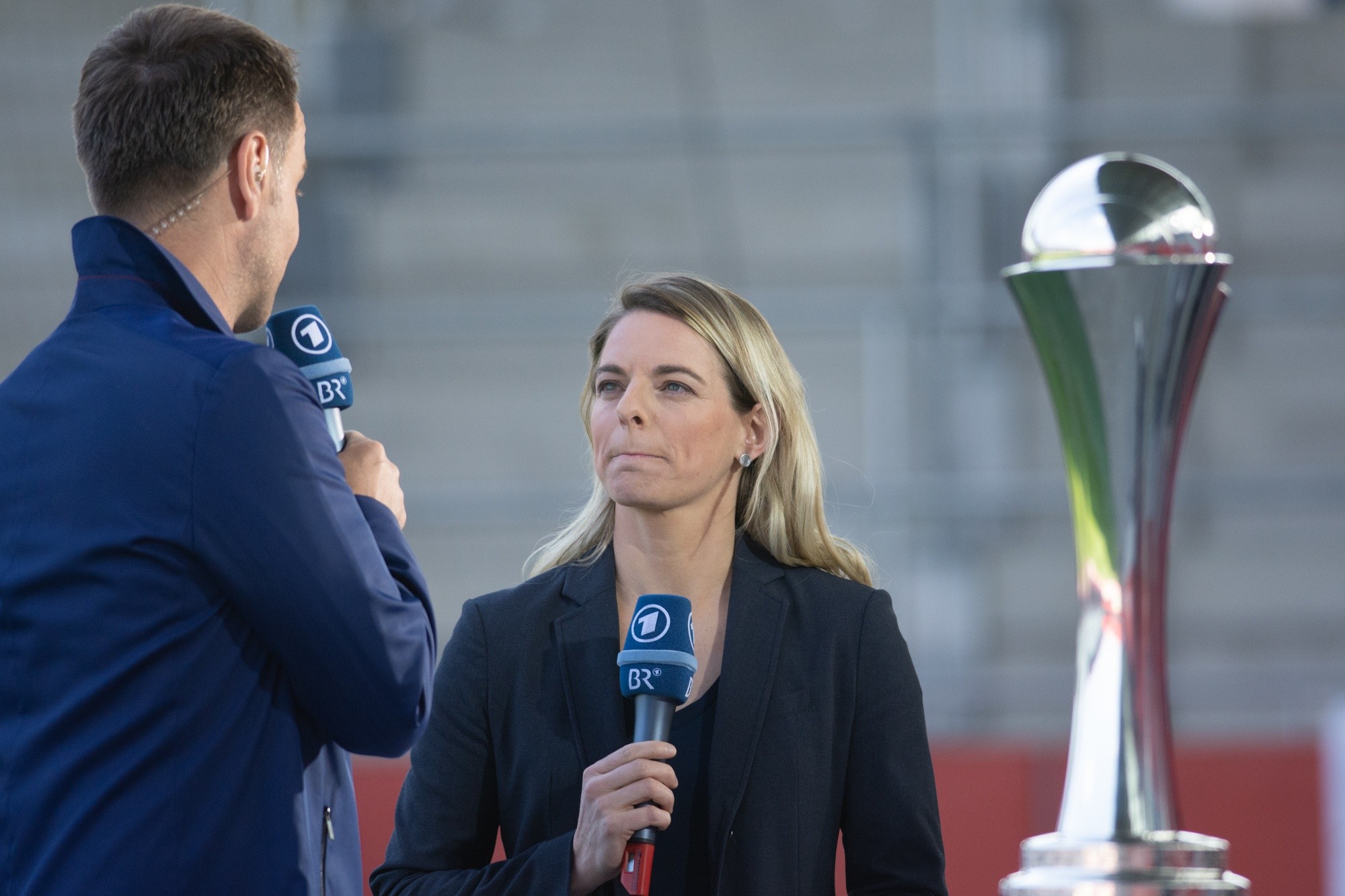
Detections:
[620,594,697,894]
[264,306,353,453]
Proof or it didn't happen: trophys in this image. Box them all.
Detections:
[999,151,1253,895]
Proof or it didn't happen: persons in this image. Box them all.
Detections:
[0,2,438,896]
[367,265,948,896]
